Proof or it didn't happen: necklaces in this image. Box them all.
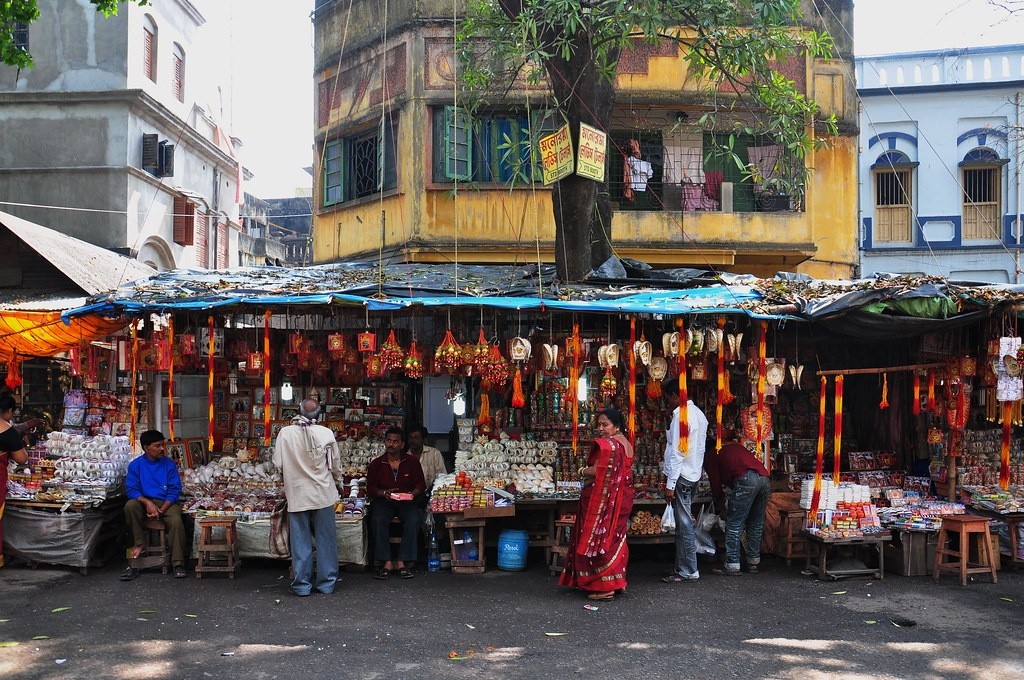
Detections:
[390,456,400,463]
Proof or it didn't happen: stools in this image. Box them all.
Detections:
[129,518,171,575]
[374,516,402,572]
[932,515,997,586]
[550,512,577,576]
[196,516,237,579]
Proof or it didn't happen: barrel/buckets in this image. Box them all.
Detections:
[498,529,528,570]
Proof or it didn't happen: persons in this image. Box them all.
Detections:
[662,378,709,583]
[273,399,343,597]
[0,395,45,569]
[557,409,634,601]
[366,425,448,580]
[703,438,771,576]
[123,430,189,578]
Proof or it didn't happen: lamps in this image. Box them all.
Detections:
[677,112,689,123]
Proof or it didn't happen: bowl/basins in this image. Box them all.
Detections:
[438,553,452,569]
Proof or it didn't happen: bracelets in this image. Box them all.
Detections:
[383,490,386,495]
[577,467,586,477]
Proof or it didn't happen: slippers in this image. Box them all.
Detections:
[662,575,698,582]
[740,561,759,574]
[587,590,615,602]
[374,567,394,579]
[712,565,742,575]
[398,567,414,578]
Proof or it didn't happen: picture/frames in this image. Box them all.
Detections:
[213,380,405,438]
[164,437,207,475]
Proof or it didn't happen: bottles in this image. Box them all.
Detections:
[428,534,440,572]
[457,531,478,559]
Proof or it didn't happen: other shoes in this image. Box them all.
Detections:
[174,566,187,577]
[119,567,137,579]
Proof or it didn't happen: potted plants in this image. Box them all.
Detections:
[740,155,795,212]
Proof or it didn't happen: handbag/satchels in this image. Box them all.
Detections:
[661,501,675,536]
[689,502,726,559]
[269,498,291,557]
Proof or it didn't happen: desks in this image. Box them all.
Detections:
[776,509,892,581]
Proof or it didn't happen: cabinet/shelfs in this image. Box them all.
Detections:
[627,497,725,544]
[0,360,64,426]
[153,374,209,443]
